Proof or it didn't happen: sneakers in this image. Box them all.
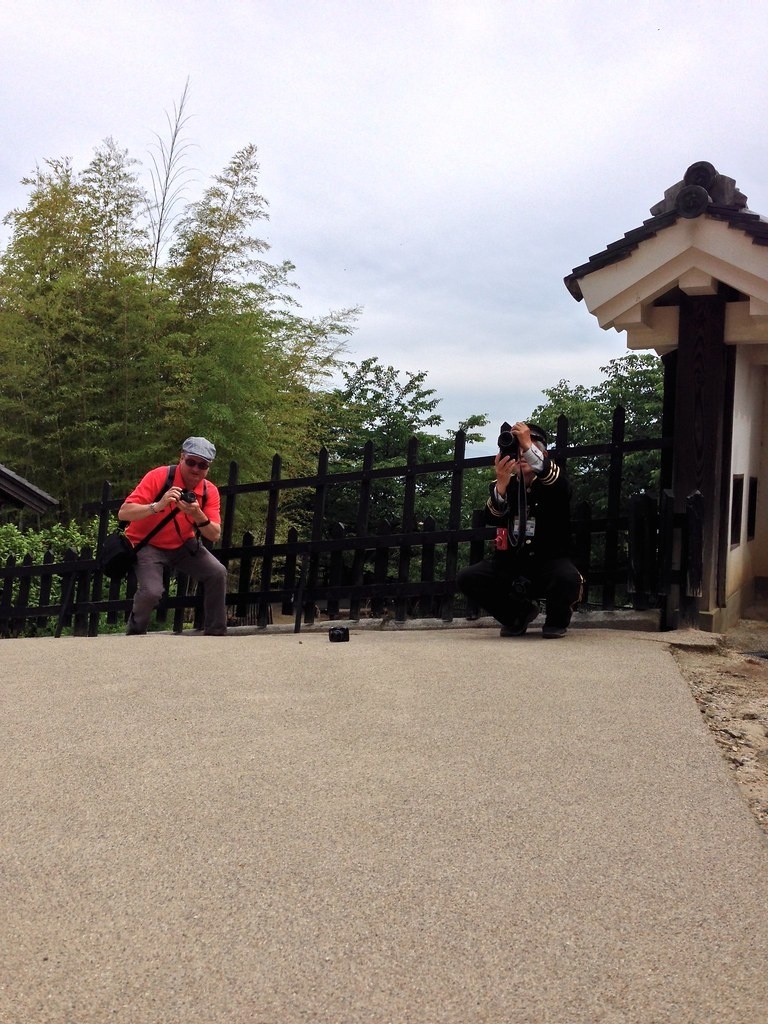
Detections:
[542,621,568,640]
[500,604,540,637]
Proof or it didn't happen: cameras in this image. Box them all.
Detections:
[179,490,196,504]
[329,626,349,642]
[498,431,519,464]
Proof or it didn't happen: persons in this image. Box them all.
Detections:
[118,437,227,636]
[457,421,586,638]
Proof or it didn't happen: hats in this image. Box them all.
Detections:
[181,436,217,464]
[526,423,547,447]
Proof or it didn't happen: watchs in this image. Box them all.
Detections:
[150,502,159,514]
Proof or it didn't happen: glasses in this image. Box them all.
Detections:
[184,458,210,470]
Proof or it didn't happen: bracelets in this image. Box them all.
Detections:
[197,518,210,527]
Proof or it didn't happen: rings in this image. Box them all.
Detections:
[522,423,525,425]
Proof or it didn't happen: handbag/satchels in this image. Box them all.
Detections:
[98,531,138,580]
